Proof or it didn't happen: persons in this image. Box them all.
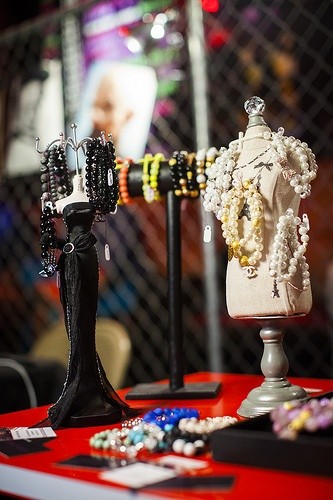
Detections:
[212,97,313,320]
[47,174,130,429]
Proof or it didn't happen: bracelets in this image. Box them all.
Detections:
[37,124,333,467]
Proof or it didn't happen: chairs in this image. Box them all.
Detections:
[0,317,132,414]
